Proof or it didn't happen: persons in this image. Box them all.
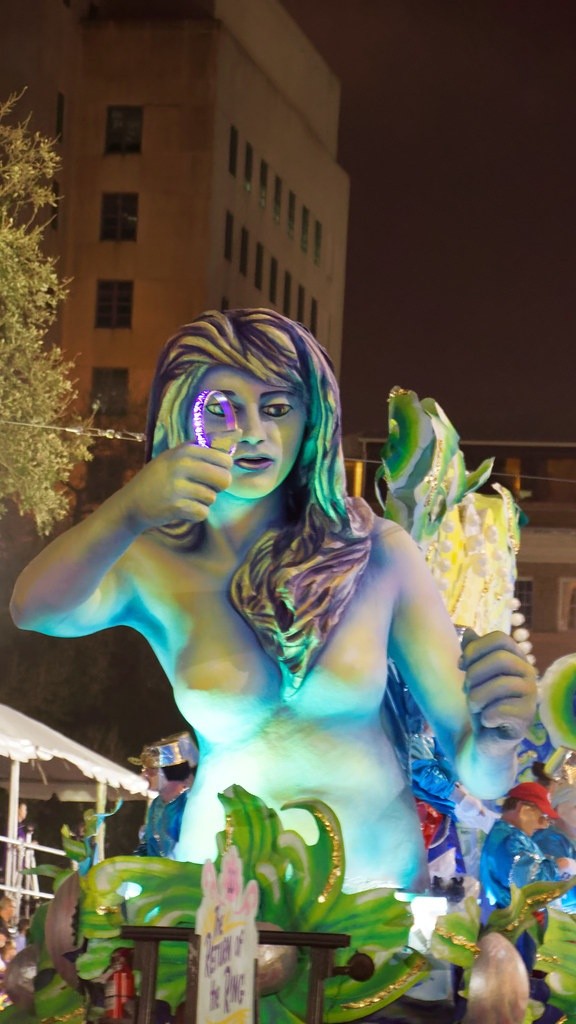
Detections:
[477,782,576,963]
[17,801,28,869]
[9,307,535,891]
[128,730,199,858]
[406,686,486,896]
[0,897,33,1010]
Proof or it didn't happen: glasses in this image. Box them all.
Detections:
[525,805,548,825]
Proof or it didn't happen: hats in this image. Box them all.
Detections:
[507,782,559,820]
[127,741,186,768]
[162,731,199,770]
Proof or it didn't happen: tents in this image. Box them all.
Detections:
[0,703,150,889]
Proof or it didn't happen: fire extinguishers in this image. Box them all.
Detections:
[103,945,136,1024]
